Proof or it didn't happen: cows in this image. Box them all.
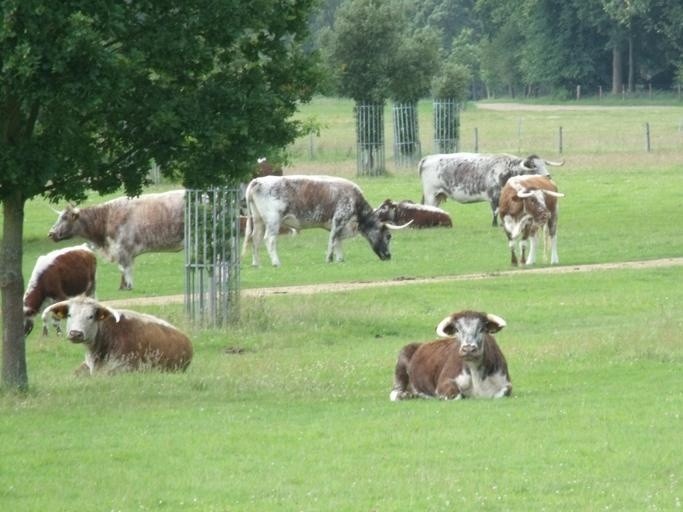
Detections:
[416,150,568,228]
[241,173,417,269]
[251,152,283,178]
[20,240,98,340]
[496,172,565,269]
[376,197,453,228]
[387,308,515,400]
[39,295,195,380]
[44,197,220,292]
